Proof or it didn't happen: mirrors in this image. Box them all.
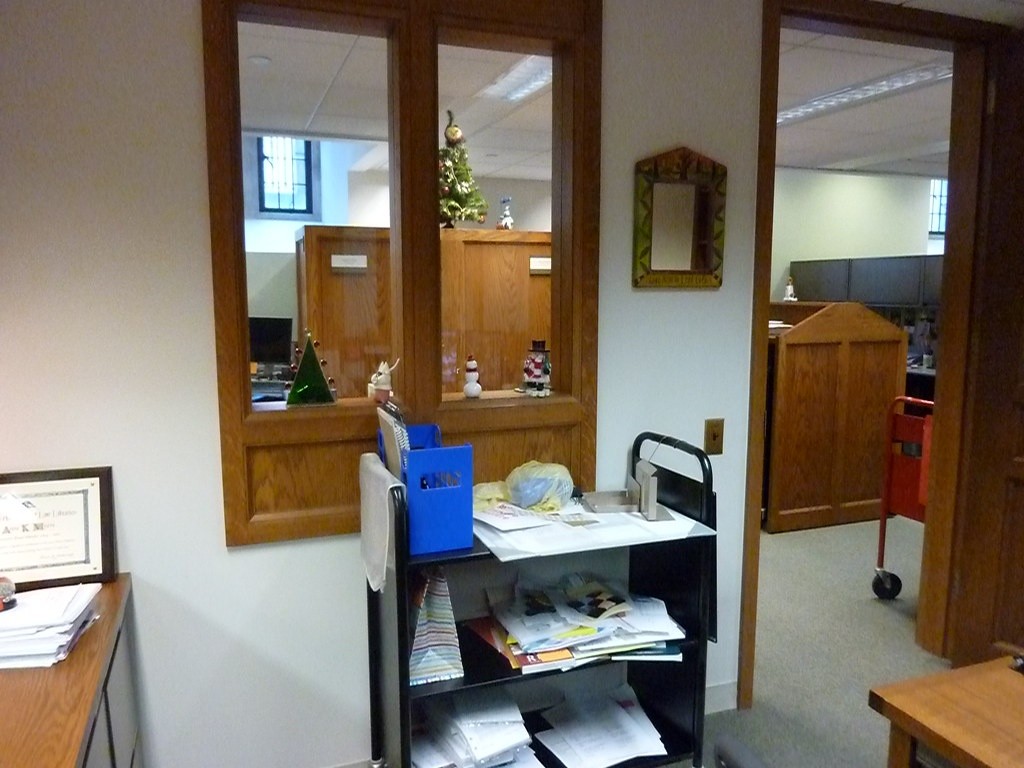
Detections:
[633,146,728,288]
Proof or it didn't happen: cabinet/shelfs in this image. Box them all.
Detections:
[762,255,944,533]
[297,225,552,399]
[360,432,712,768]
[0,573,144,768]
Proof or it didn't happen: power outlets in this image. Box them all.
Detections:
[705,418,723,455]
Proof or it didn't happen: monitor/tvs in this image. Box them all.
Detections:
[247,316,292,378]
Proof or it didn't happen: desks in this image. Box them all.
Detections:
[868,656,1024,768]
